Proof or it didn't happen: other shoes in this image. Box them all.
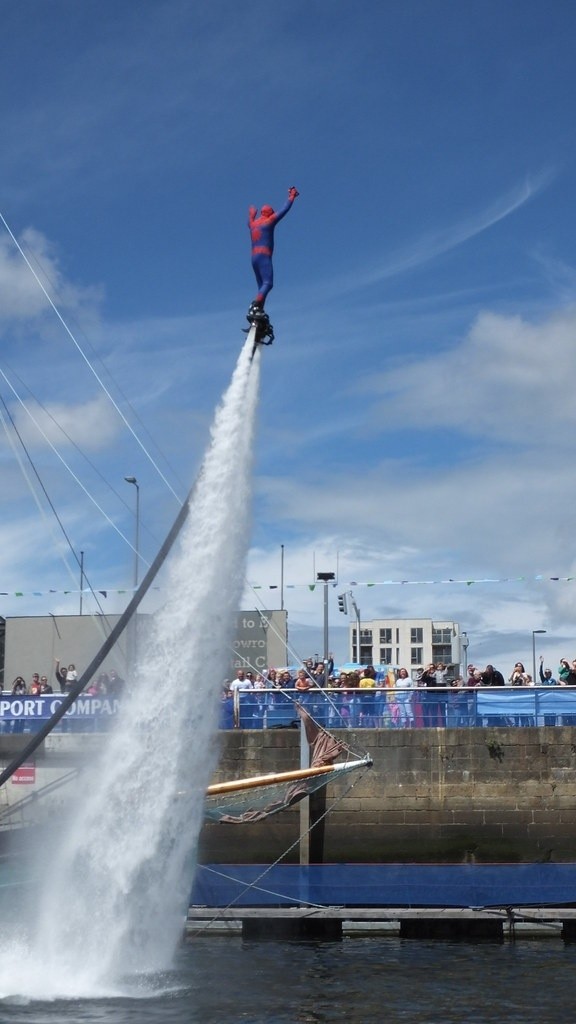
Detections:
[248,302,269,318]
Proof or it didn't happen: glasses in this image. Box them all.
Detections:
[33,676,39,678]
[247,675,252,678]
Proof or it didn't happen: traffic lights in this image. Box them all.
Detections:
[338,593,346,615]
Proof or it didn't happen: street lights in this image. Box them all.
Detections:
[532,628,546,726]
[123,474,141,693]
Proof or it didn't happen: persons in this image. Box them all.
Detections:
[248,186,297,309]
[220,656,576,728]
[11,657,125,696]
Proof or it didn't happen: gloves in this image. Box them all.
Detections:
[289,189,297,202]
[250,208,257,216]
